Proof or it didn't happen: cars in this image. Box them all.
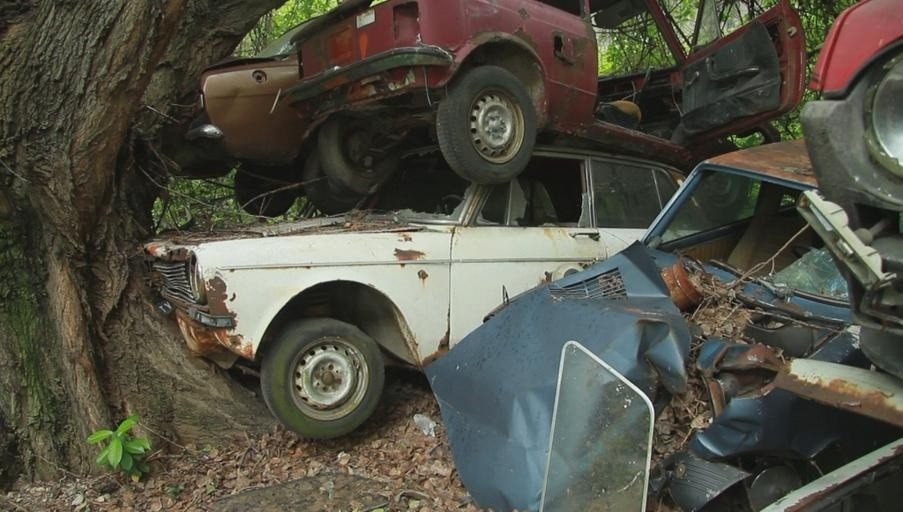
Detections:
[145,0,903,511]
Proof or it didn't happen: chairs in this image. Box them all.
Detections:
[483,179,558,226]
